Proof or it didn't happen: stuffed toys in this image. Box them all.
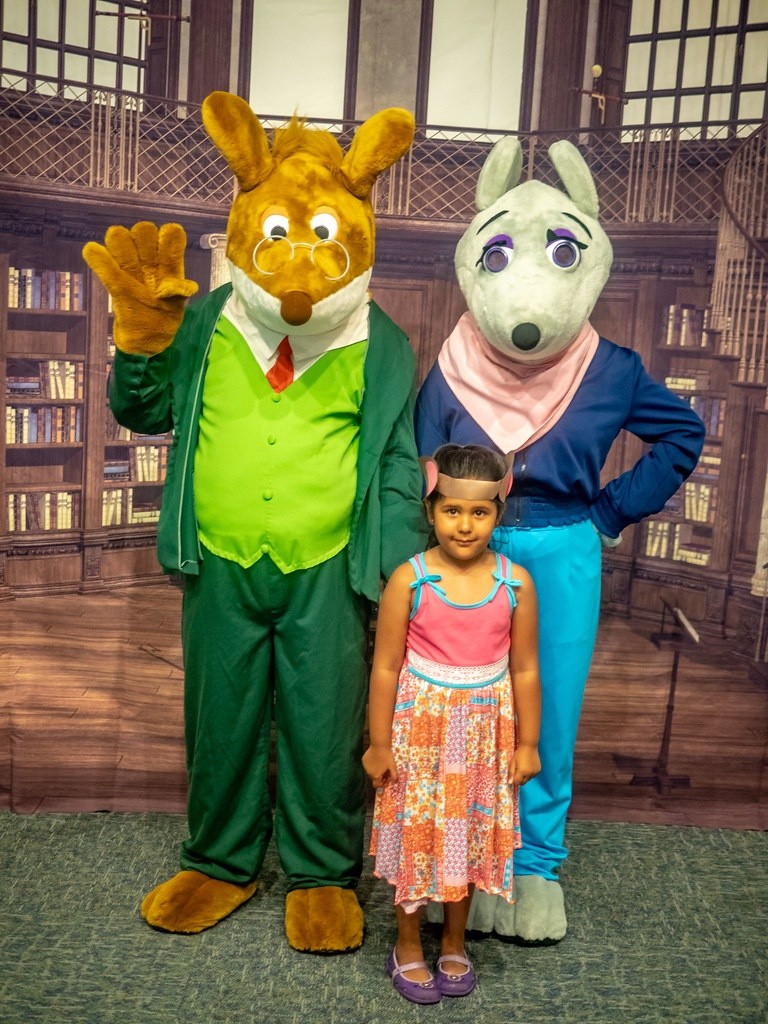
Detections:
[415,138,706,939]
[80,90,432,951]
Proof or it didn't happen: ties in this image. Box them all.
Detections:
[266,336,295,393]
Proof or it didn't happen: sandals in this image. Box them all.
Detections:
[435,950,477,997]
[386,944,441,1004]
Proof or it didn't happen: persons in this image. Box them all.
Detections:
[359,446,541,1005]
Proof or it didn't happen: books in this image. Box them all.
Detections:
[643,301,730,563]
[6,266,172,535]
